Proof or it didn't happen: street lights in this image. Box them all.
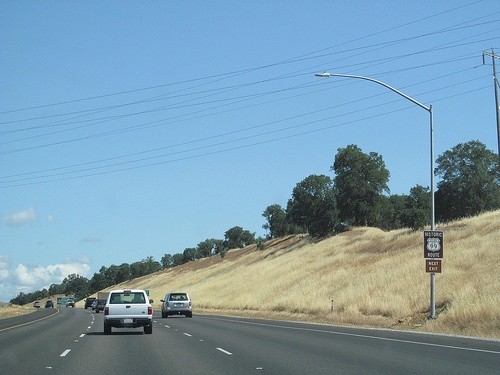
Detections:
[313,70,437,320]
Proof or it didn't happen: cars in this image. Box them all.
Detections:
[45,300,54,308]
[33,301,40,308]
[65,300,75,308]
[91,298,107,313]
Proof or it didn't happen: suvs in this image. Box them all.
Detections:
[160,291,193,318]
[102,289,154,334]
[83,297,97,309]
